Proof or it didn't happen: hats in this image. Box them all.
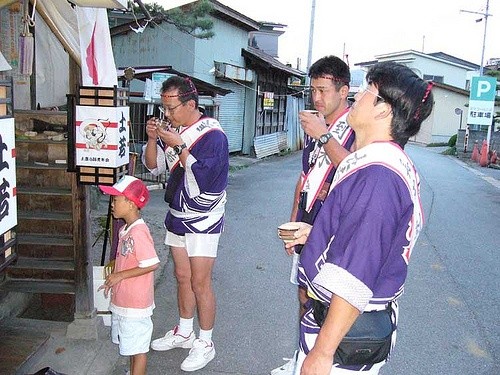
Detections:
[98,175,151,209]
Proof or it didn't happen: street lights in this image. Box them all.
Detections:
[460,0,494,76]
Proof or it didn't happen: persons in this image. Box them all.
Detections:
[98,176,161,375]
[270,54,356,375]
[285,61,432,375]
[142,76,228,371]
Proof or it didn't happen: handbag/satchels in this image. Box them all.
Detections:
[311,298,398,366]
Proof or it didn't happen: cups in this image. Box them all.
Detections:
[156,121,171,131]
[279,228,298,243]
[303,110,318,116]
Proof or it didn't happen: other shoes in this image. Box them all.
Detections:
[270,350,298,375]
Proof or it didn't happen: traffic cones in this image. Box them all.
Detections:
[472,139,497,166]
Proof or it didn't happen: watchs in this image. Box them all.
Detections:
[318,133,333,147]
[173,143,186,155]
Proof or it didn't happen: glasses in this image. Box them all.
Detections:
[159,103,184,116]
[358,84,385,102]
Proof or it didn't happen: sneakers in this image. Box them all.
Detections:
[151,325,196,351]
[181,336,215,371]
